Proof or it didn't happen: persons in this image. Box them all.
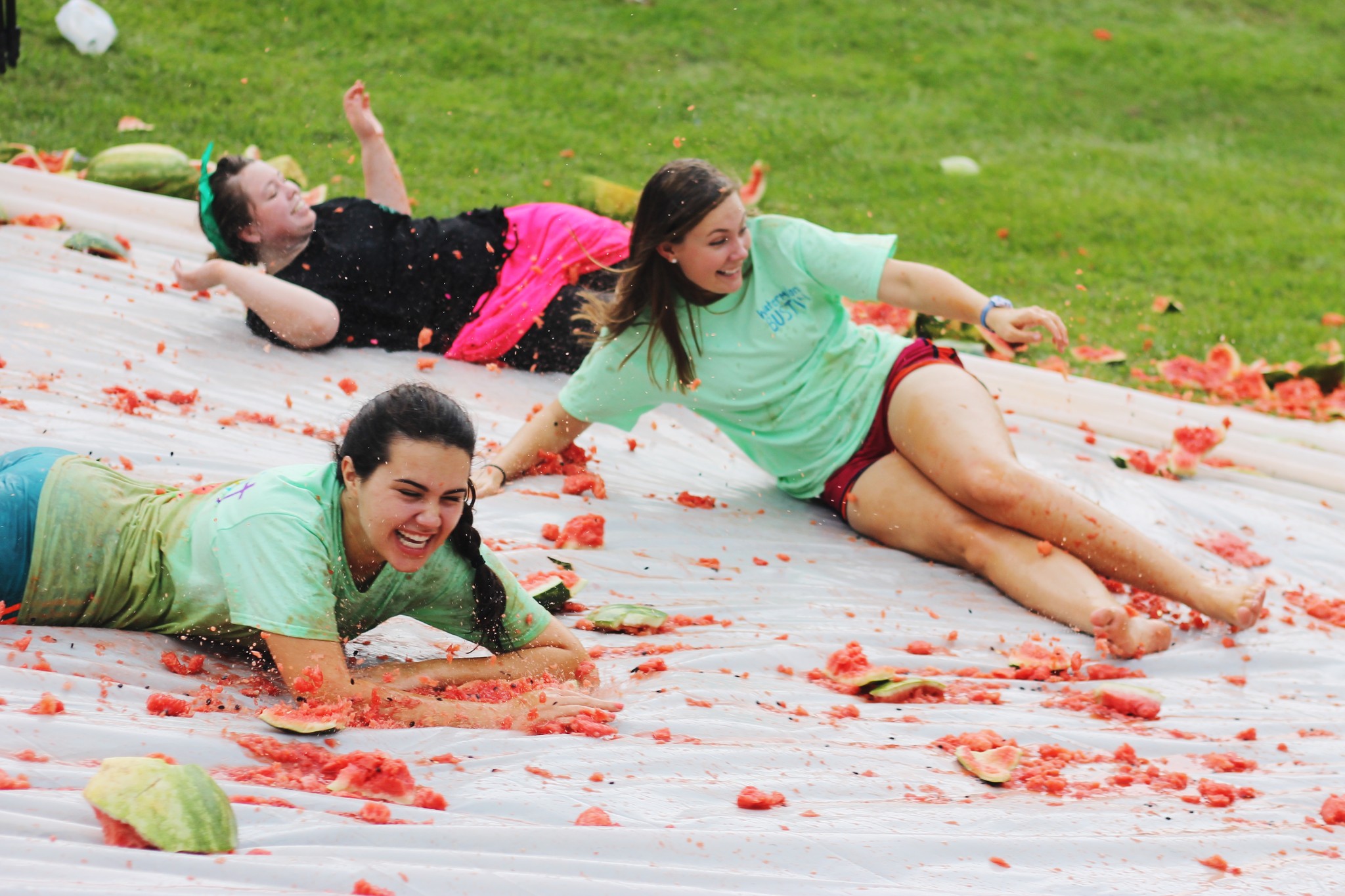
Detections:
[467,158,1266,657]
[0,384,623,734]
[176,81,633,374]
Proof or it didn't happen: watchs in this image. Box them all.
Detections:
[981,294,1013,333]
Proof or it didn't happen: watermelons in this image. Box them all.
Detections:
[0,143,1344,854]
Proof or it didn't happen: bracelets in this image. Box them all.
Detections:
[480,465,506,487]
